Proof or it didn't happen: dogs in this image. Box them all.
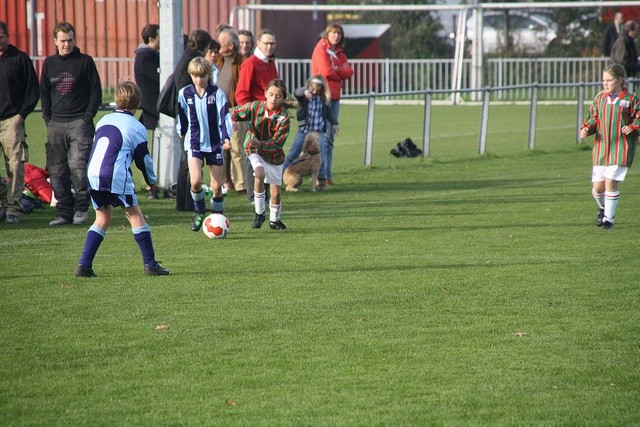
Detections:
[282,130,323,193]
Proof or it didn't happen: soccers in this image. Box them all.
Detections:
[202,213,230,239]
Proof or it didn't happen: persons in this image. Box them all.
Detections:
[600,12,625,57]
[152,30,211,197]
[40,21,102,226]
[133,25,160,128]
[0,22,40,224]
[311,24,354,191]
[282,73,340,191]
[578,64,640,229]
[214,28,246,194]
[611,20,640,77]
[237,30,252,59]
[202,39,221,84]
[75,80,168,276]
[235,30,279,106]
[213,23,228,43]
[176,56,232,231]
[230,79,298,230]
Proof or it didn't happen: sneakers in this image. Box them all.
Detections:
[596,207,604,225]
[251,212,266,229]
[162,190,174,198]
[7,215,20,224]
[144,261,169,276]
[269,220,287,231]
[0,208,5,220]
[49,216,70,227]
[73,212,88,225]
[148,188,160,199]
[75,263,98,278]
[601,220,613,229]
[318,184,330,192]
[248,196,255,207]
[191,209,206,231]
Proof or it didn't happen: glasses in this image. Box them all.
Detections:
[260,39,277,46]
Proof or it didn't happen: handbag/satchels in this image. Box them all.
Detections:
[156,73,177,118]
